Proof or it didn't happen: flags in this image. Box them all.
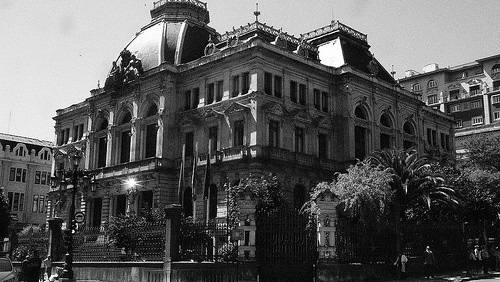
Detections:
[178,144,185,195]
[203,139,210,200]
[191,144,198,204]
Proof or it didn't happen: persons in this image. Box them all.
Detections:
[20,253,53,282]
[468,245,499,273]
[393,252,408,282]
[125,55,143,83]
[102,61,122,94]
[422,246,437,280]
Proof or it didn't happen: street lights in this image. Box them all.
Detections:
[64,152,82,282]
